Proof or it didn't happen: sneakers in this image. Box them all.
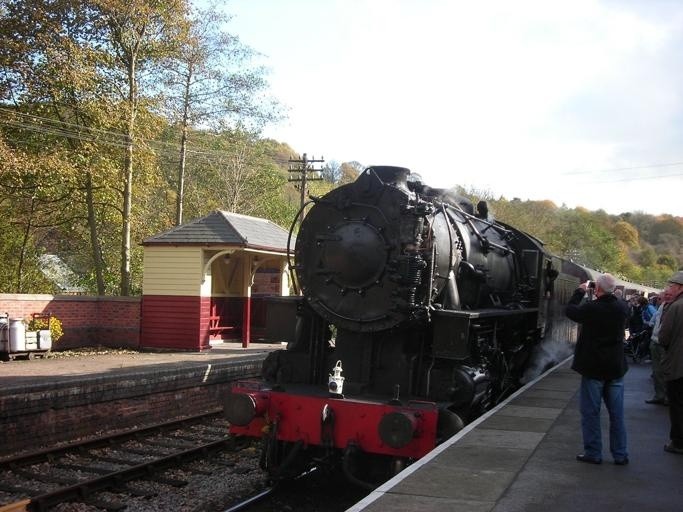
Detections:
[663,444,683,454]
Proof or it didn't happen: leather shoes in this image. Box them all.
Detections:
[576,454,602,464]
[615,458,629,465]
[645,398,664,404]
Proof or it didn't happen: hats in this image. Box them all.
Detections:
[668,270,683,285]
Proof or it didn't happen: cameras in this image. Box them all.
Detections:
[585,280,595,289]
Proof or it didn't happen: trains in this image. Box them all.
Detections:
[217,162,669,477]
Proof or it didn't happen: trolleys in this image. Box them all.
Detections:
[0,312,52,361]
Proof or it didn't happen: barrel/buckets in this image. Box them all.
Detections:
[6,318,25,351]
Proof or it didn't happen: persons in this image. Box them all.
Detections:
[564,273,629,465]
[656,270,682,455]
[644,300,671,406]
[614,287,664,379]
